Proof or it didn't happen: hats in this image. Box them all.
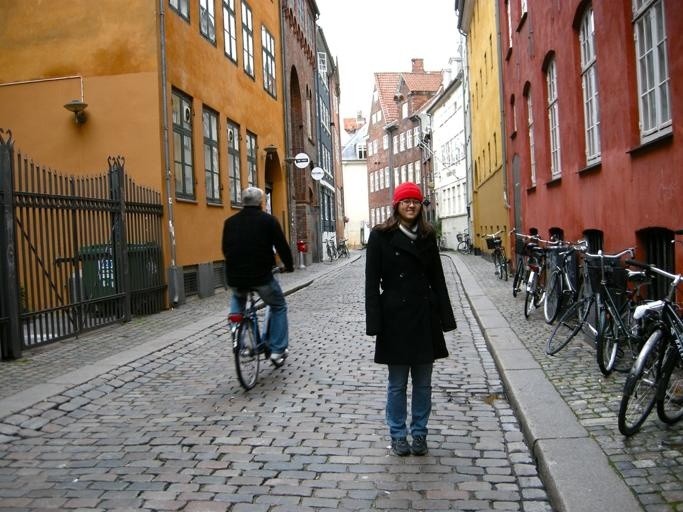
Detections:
[392,181,423,207]
[240,186,264,207]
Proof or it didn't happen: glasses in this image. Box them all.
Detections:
[398,199,421,207]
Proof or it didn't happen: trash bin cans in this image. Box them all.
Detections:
[475,249,480,255]
[80,244,162,317]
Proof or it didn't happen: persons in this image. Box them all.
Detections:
[222,188,294,366]
[365,183,457,456]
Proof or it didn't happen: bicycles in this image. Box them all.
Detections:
[509,226,683,435]
[479,230,512,281]
[325,236,350,262]
[456,227,472,255]
[228,265,287,390]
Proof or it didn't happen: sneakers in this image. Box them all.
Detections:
[410,434,428,456]
[390,435,411,456]
[269,348,289,361]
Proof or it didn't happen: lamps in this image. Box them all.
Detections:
[63,98,88,124]
